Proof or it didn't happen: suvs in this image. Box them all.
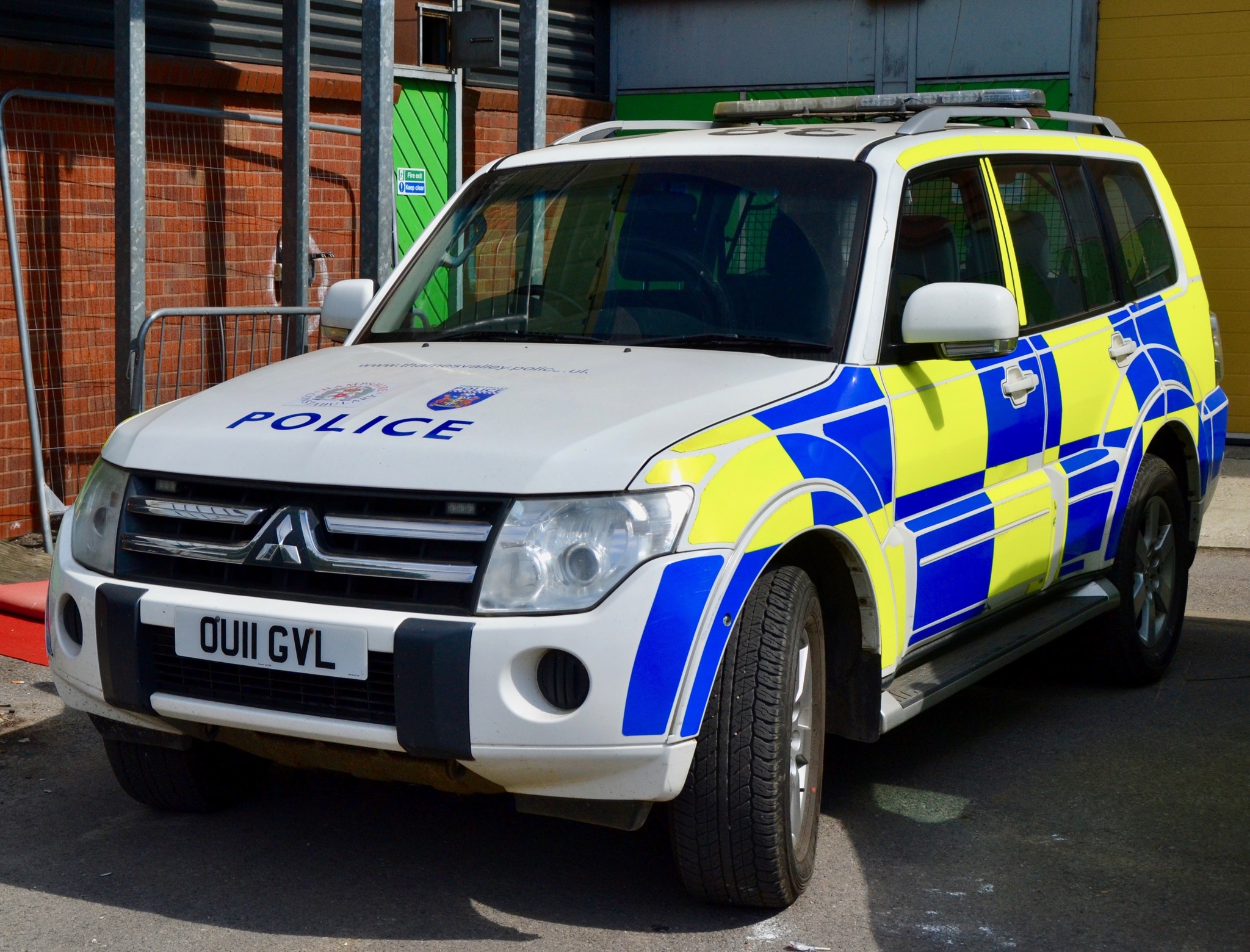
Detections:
[47,87,1227,916]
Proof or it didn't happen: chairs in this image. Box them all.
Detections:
[966,211,1058,327]
[887,216,960,326]
[585,191,697,312]
[763,205,841,344]
